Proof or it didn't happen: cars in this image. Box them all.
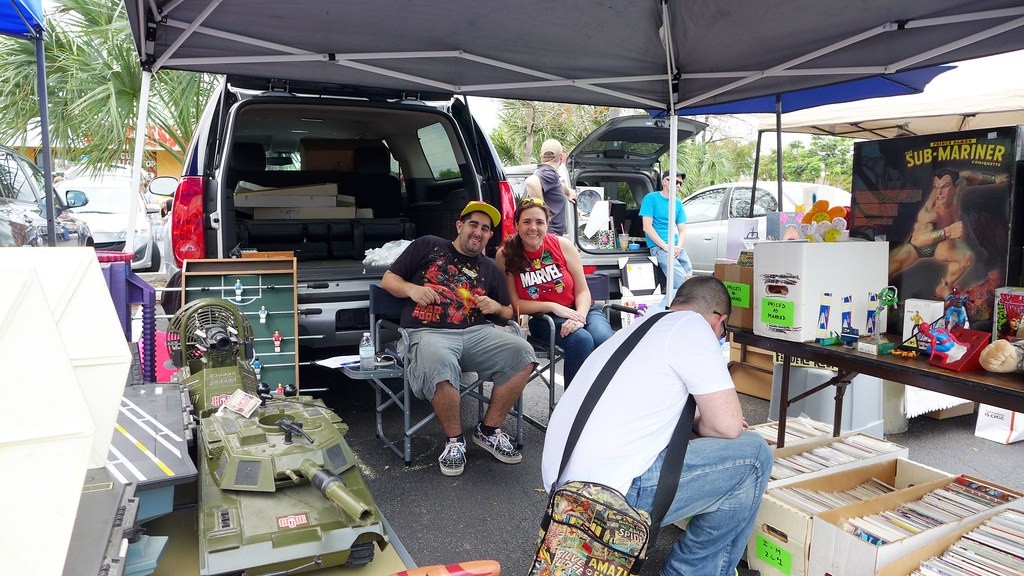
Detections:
[676,177,852,279]
[52,160,163,274]
[0,141,96,248]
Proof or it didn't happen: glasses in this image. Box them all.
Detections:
[521,198,545,207]
[667,178,682,186]
[713,311,726,341]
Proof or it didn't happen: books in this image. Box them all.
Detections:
[766,475,899,517]
[905,508,1024,576]
[751,417,832,444]
[773,433,906,480]
[838,476,1022,545]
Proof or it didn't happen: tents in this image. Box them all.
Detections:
[0,1,56,246]
[123,1,1024,311]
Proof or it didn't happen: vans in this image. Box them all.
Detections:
[501,110,710,306]
[147,70,523,392]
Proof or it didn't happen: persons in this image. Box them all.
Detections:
[917,322,962,364]
[140,181,161,204]
[937,286,975,330]
[381,200,540,476]
[542,277,774,576]
[636,170,692,289]
[495,198,616,393]
[526,139,578,236]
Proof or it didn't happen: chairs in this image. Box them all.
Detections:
[369,284,646,467]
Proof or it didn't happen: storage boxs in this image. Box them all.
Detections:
[233,181,374,220]
[713,239,1024,575]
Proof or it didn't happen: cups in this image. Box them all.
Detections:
[617,233,629,249]
[513,328,528,341]
[629,244,640,250]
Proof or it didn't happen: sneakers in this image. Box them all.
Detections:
[471,423,522,464]
[438,436,467,475]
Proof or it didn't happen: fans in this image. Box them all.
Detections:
[166,297,254,376]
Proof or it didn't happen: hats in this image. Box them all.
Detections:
[541,139,562,158]
[460,200,501,231]
[662,169,686,180]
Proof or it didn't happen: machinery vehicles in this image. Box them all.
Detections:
[193,391,388,576]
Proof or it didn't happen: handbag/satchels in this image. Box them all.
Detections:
[526,483,652,576]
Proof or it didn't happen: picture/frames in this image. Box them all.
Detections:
[618,257,660,295]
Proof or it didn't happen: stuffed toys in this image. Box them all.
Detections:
[978,339,1024,374]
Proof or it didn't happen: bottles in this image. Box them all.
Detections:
[358,332,375,371]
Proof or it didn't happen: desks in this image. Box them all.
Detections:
[732,333,1024,449]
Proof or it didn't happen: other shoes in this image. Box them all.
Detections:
[735,566,760,576]
